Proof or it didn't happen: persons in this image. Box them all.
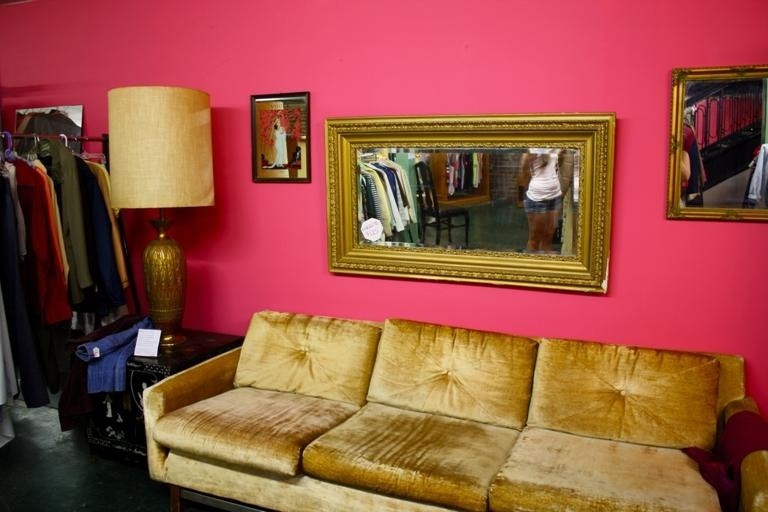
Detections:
[679,97,705,209]
[517,147,566,252]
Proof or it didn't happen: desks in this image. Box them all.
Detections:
[74,327,243,469]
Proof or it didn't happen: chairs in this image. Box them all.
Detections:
[415,163,469,248]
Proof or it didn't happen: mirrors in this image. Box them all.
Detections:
[326,112,616,295]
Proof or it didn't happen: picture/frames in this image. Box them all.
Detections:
[665,63,768,223]
[250,91,312,183]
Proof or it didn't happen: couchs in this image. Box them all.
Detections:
[142,308,767,512]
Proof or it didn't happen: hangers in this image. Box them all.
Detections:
[1,131,106,169]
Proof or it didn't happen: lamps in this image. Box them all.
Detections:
[108,86,215,345]
